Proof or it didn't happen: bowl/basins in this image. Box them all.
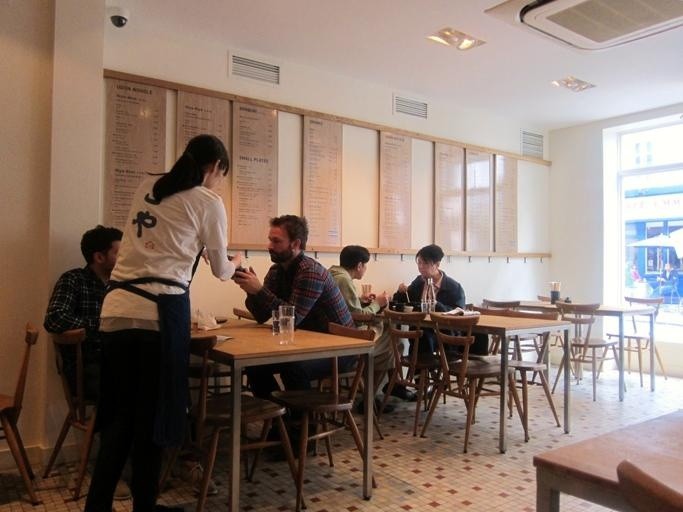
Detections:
[388,300,421,311]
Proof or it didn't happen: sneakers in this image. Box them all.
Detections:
[179,464,218,495]
[359,399,394,414]
[382,383,414,400]
[113,481,131,499]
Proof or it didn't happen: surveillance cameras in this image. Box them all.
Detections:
[105,7,131,27]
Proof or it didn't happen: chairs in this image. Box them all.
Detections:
[616,459,683,512]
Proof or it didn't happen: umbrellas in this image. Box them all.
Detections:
[627,232,673,292]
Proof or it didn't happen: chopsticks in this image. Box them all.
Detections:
[550,281,561,293]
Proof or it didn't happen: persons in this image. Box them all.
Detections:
[230,214,358,461]
[42,221,219,495]
[393,244,467,402]
[625,261,679,303]
[83,132,244,512]
[324,246,418,413]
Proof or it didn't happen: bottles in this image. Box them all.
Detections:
[424,277,436,317]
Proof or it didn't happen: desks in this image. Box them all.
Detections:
[532,409,683,512]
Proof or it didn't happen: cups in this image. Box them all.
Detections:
[550,291,559,305]
[360,284,371,302]
[270,304,295,346]
